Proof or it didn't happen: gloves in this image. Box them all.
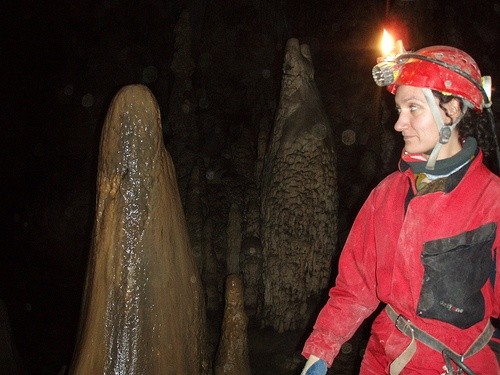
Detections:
[300,355,327,375]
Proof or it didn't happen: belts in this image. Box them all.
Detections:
[385,304,494,375]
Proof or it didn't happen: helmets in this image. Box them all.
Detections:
[386,46,484,113]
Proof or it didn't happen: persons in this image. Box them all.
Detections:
[301,45,500,375]
